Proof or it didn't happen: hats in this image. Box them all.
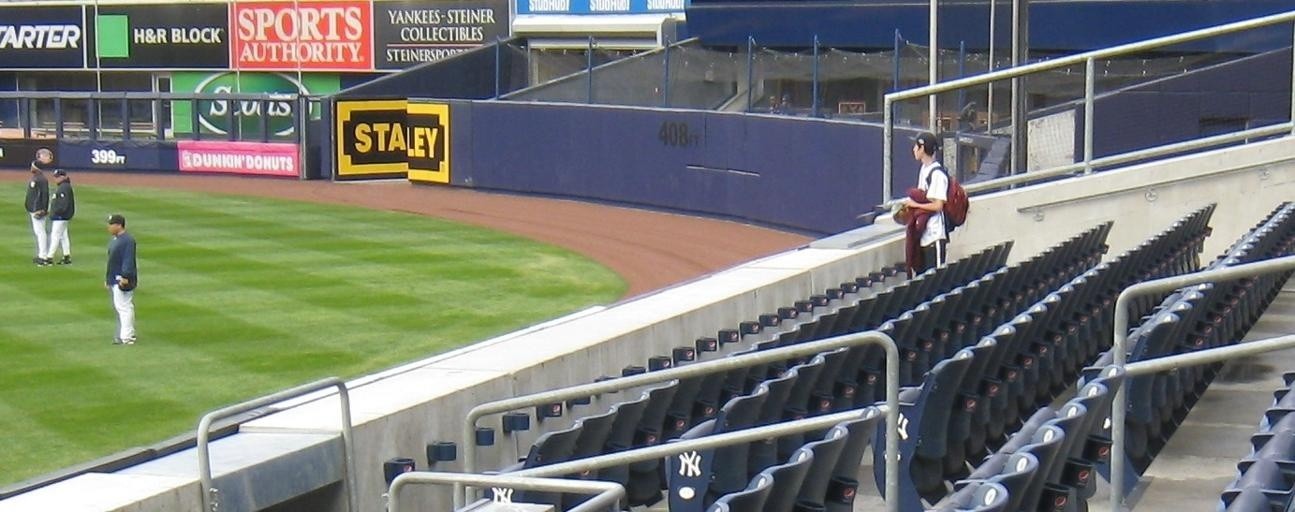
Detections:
[52,169,66,177]
[106,214,126,226]
[908,131,940,153]
[30,160,46,173]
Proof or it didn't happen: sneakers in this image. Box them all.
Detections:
[111,334,137,345]
[33,255,72,267]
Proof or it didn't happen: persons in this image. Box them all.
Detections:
[103,214,138,345]
[888,130,951,278]
[24,160,50,265]
[768,94,797,118]
[34,168,75,266]
[959,101,980,132]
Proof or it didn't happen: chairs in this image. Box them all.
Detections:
[480,199,1295,512]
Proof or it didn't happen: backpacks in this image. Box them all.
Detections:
[926,167,969,226]
